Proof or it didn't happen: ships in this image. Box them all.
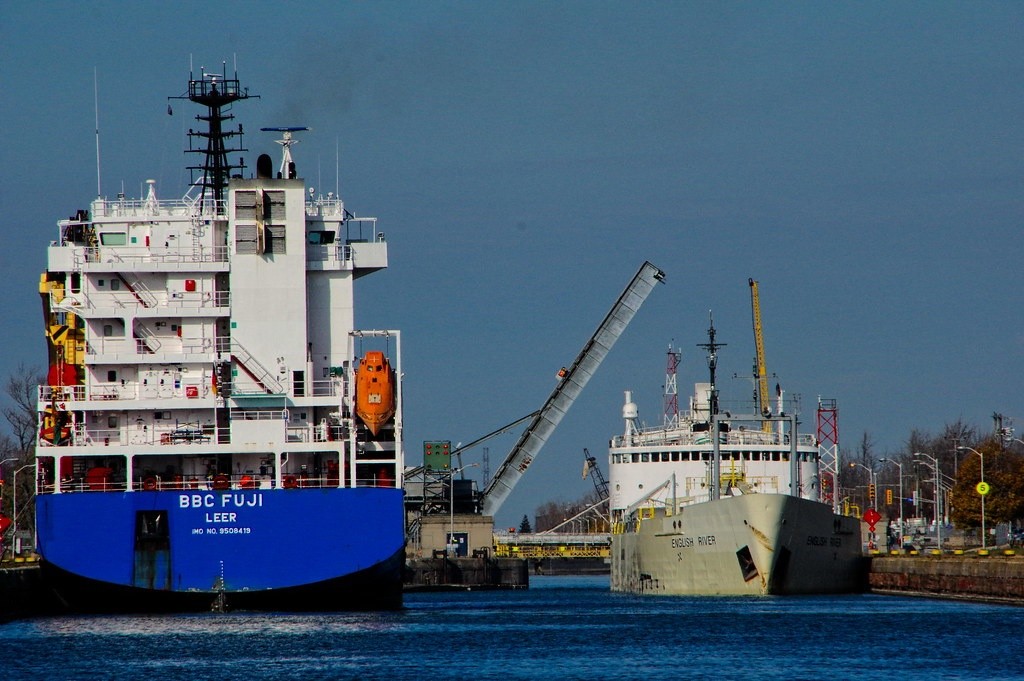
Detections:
[32,51,407,615]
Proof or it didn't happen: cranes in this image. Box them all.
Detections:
[748,278,773,432]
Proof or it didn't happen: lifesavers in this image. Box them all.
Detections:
[144,476,157,490]
[283,475,297,488]
[213,476,228,490]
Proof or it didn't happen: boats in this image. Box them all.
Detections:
[403,439,530,592]
[607,308,874,598]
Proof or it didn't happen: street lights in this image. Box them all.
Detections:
[958,446,986,550]
[914,453,957,549]
[12,464,36,559]
[879,458,903,549]
[851,463,873,505]
[563,516,597,535]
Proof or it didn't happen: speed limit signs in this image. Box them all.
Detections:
[977,482,990,495]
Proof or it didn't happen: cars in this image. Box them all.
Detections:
[1007,528,1024,546]
[2,549,41,564]
[924,546,938,557]
